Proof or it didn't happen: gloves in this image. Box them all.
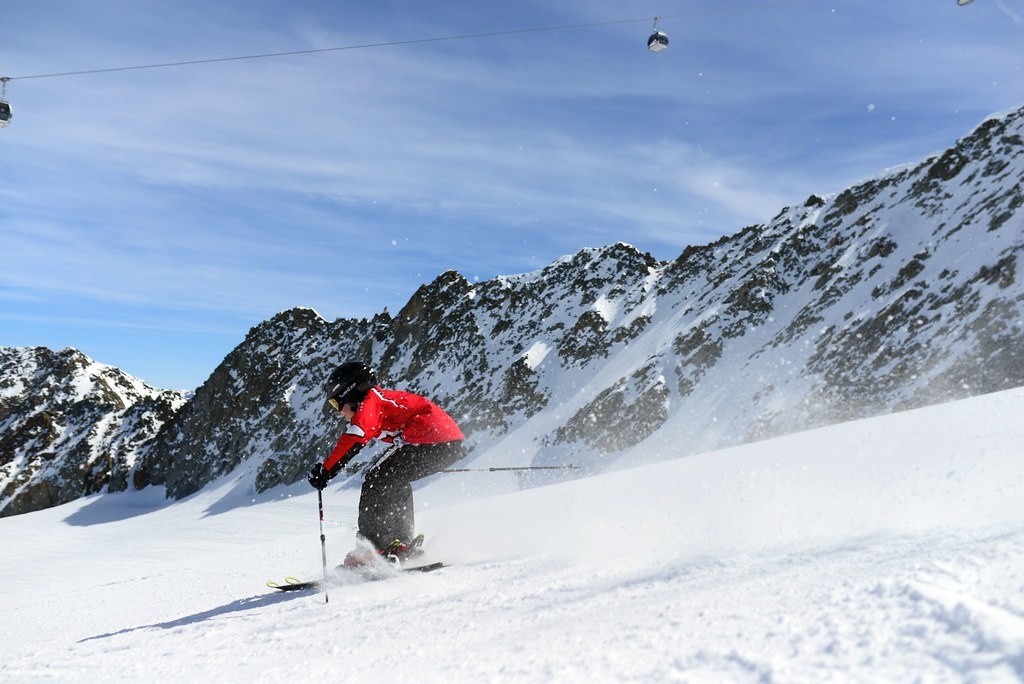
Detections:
[308,462,333,490]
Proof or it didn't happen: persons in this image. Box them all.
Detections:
[308,360,467,565]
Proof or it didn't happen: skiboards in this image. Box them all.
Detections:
[265,560,446,593]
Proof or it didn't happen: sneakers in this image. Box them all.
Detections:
[341,534,423,567]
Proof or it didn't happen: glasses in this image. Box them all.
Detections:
[326,396,344,412]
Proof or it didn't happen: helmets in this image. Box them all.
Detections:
[323,362,378,422]
